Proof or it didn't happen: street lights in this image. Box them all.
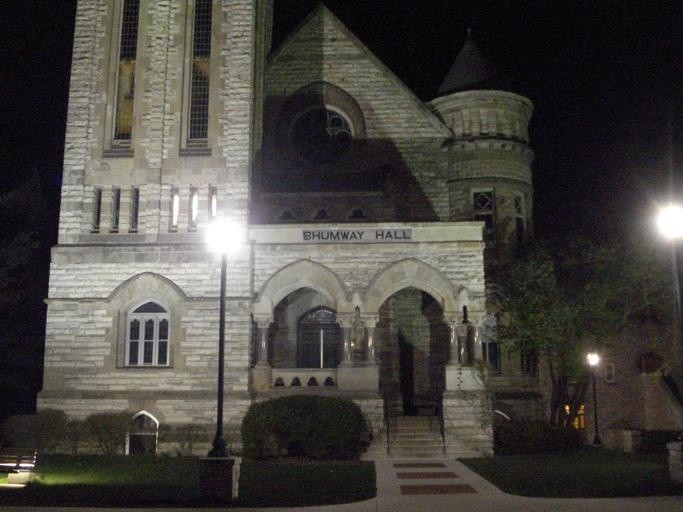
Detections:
[202,214,239,460]
[583,353,602,446]
[658,204,683,302]
